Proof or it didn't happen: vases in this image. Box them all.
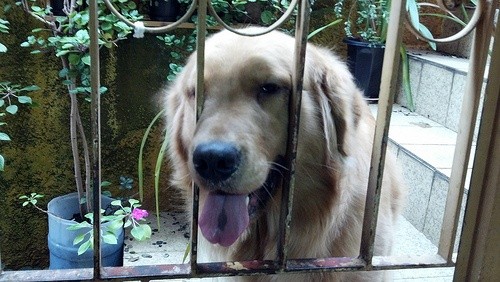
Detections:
[47,193,124,269]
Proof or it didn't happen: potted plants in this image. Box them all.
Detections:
[334,0,391,103]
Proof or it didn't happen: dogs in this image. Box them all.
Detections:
[160,26,405,282]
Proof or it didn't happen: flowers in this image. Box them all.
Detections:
[15,0,152,254]
